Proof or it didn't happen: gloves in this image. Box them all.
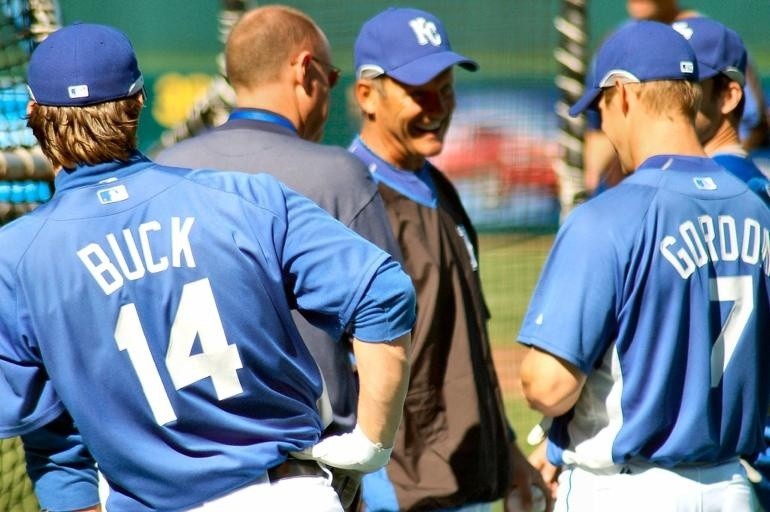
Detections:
[288,429,394,510]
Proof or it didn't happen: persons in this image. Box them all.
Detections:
[583,0,765,191]
[669,14,768,203]
[0,18,417,512]
[517,16,768,512]
[344,5,552,512]
[149,4,405,512]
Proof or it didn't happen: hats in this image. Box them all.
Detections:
[25,22,145,108]
[568,21,700,120]
[354,6,480,88]
[668,17,747,89]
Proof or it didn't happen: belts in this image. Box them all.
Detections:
[266,458,329,484]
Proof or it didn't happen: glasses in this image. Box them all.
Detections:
[291,54,342,90]
